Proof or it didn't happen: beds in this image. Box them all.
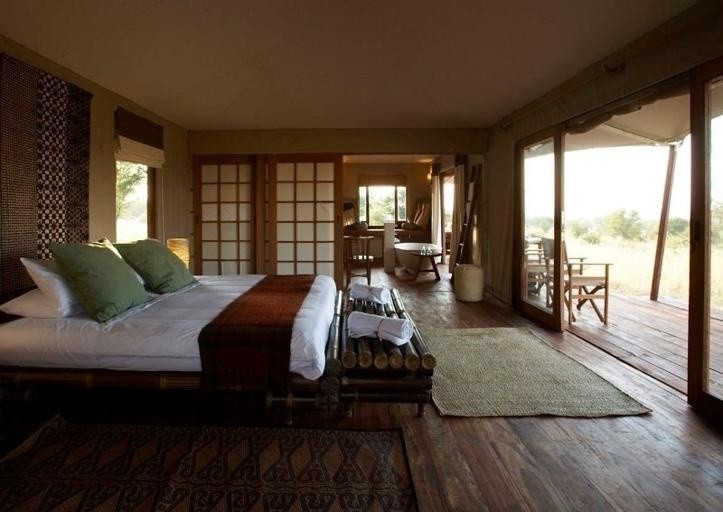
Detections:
[0,275,437,423]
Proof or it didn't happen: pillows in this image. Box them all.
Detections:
[19,256,145,316]
[47,236,155,323]
[0,289,82,319]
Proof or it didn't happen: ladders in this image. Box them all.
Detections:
[450,164,480,292]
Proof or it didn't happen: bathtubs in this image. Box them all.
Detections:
[394,242,442,272]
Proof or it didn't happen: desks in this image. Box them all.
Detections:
[411,253,442,281]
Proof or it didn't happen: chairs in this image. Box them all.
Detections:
[525,239,588,297]
[541,237,614,326]
[343,236,375,289]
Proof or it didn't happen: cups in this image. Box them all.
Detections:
[420,244,426,255]
[426,244,432,256]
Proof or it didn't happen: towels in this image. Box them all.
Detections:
[347,310,414,346]
[351,284,391,305]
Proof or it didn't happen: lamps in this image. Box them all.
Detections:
[110,238,199,295]
[166,239,190,276]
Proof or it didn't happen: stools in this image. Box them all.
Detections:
[453,264,484,302]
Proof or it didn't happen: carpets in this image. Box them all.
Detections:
[0,419,419,512]
[418,326,652,419]
